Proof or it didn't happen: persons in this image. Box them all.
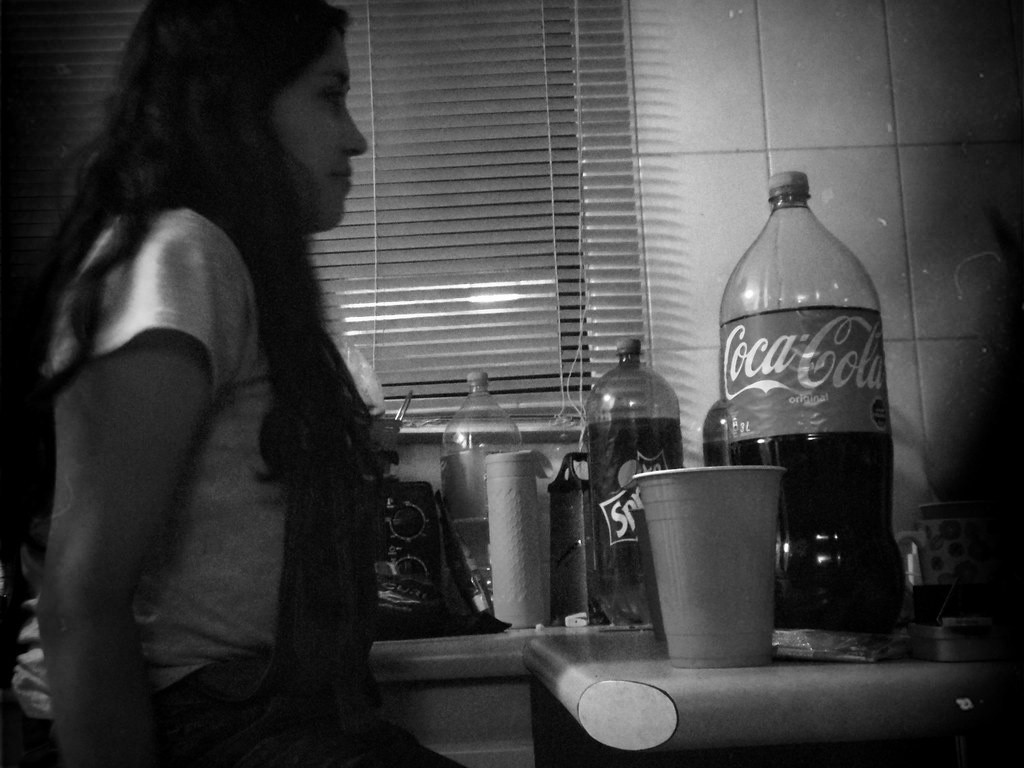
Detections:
[38,0,466,768]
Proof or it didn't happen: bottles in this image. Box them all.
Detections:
[585,338,684,627]
[702,401,731,467]
[440,370,523,622]
[547,451,587,623]
[720,171,905,634]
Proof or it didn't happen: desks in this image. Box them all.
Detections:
[369,626,655,767]
[523,629,1024,768]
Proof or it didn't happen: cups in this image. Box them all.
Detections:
[484,449,549,628]
[894,500,1008,587]
[630,464,787,669]
